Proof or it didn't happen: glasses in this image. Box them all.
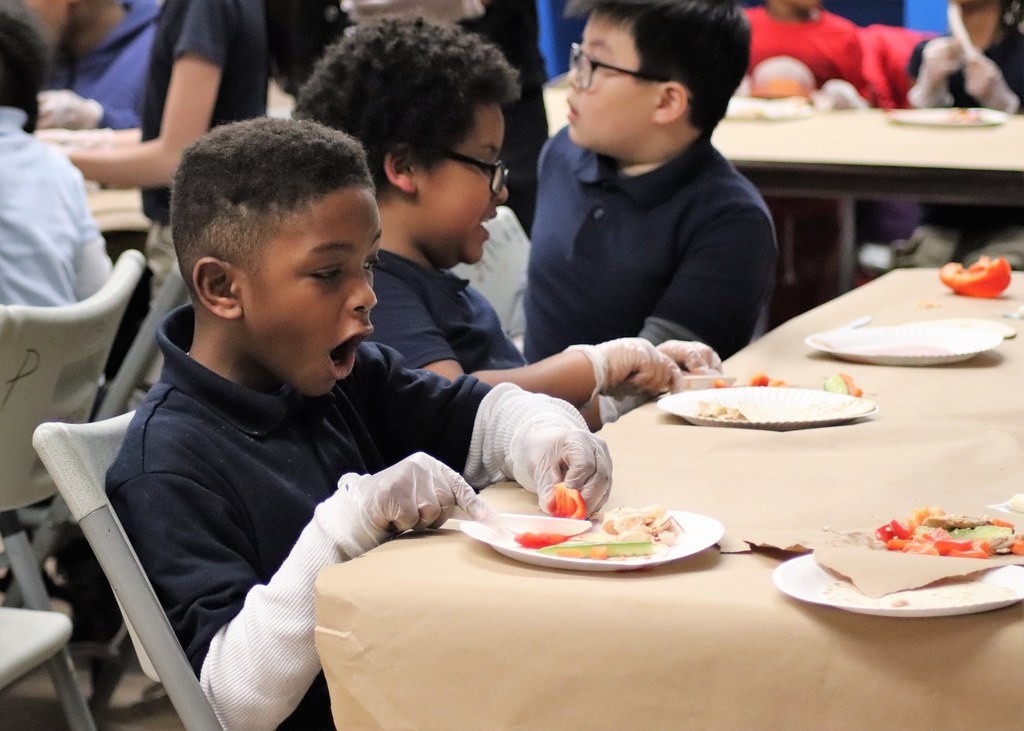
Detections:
[445,151,510,195]
[568,43,665,91]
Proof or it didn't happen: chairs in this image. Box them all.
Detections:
[0,249,148,731]
[32,406,224,731]
[0,259,190,608]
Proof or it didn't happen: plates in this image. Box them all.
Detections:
[771,553,1024,617]
[803,319,1004,366]
[887,108,1010,128]
[657,387,879,432]
[461,510,725,572]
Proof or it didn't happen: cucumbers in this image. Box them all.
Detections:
[918,526,1013,540]
[538,542,653,559]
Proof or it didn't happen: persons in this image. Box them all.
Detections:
[288,17,725,433]
[0,0,1024,407]
[525,1,776,389]
[0,14,127,709]
[102,116,613,731]
[270,1,548,242]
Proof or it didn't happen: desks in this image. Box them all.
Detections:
[313,266,1024,731]
[543,67,1024,296]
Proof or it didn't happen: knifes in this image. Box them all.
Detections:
[675,376,737,390]
[447,505,593,538]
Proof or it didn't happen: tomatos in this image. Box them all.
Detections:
[941,258,1011,296]
[514,487,584,546]
[716,374,771,387]
